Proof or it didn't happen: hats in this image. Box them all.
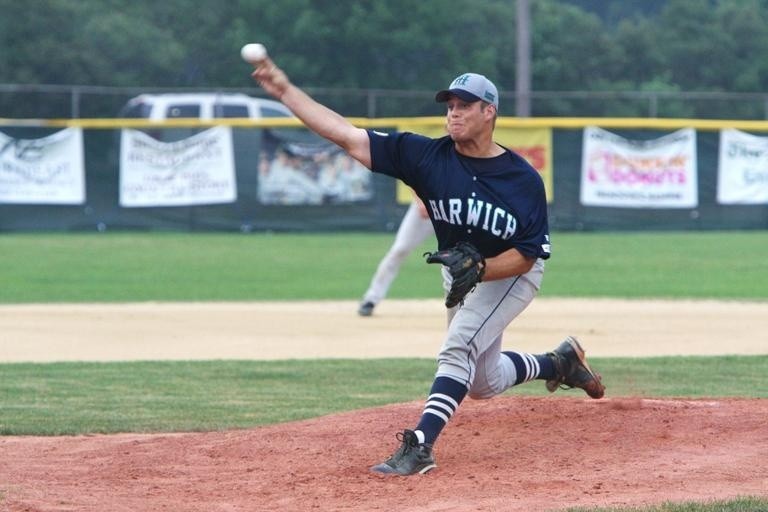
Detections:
[435,73,499,112]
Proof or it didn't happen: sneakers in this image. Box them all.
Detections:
[359,302,374,316]
[370,429,437,475]
[545,336,606,399]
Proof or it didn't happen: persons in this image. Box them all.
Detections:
[251,53,604,476]
[358,183,460,328]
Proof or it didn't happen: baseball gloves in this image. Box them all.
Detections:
[427,242,486,308]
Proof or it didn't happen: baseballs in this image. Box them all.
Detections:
[241,43,267,63]
